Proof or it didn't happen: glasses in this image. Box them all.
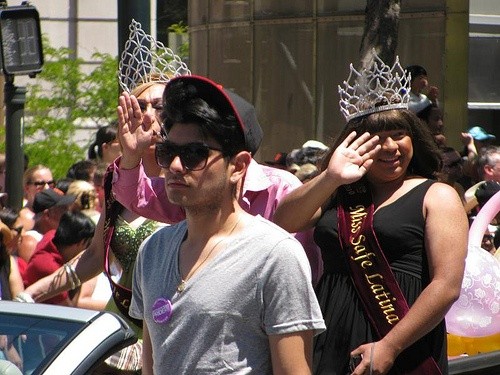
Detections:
[10,226,24,234]
[27,180,54,185]
[155,143,222,170]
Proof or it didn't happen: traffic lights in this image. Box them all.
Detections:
[1,4,46,75]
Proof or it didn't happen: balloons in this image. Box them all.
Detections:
[468,191,500,247]
[446,244,500,335]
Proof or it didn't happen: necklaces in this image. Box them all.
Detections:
[178,221,239,291]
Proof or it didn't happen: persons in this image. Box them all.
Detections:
[113,88,324,291]
[128,76,327,375]
[402,66,500,254]
[272,47,469,375]
[14,18,191,369]
[0,127,143,368]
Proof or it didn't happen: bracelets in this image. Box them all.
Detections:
[16,297,24,302]
[64,264,75,289]
[66,261,81,287]
[15,293,34,302]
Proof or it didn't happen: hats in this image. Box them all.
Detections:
[33,191,78,213]
[163,76,263,156]
[469,127,496,141]
